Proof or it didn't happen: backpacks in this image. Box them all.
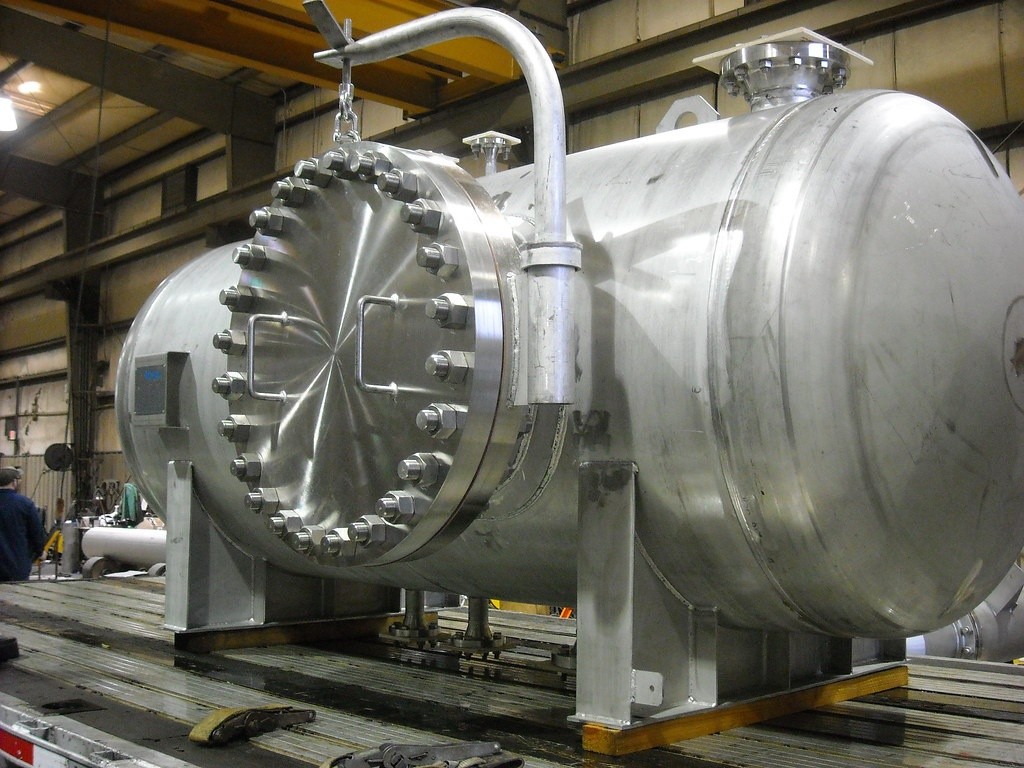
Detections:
[113,483,144,528]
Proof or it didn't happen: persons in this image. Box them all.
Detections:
[0,466,44,579]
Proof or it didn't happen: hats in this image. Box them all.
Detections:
[0,466,24,486]
